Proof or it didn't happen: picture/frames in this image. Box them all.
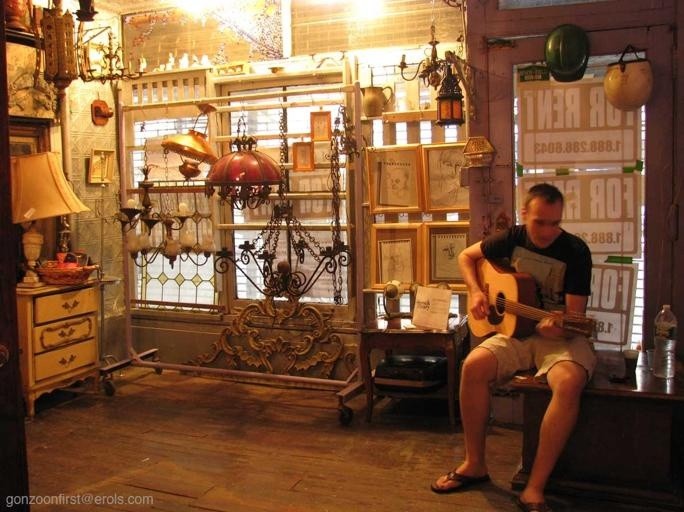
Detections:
[121,0,283,75]
[310,112,332,141]
[422,142,469,213]
[292,142,314,170]
[87,148,114,183]
[421,222,470,291]
[88,42,113,78]
[364,144,422,215]
[5,30,60,125]
[370,223,420,289]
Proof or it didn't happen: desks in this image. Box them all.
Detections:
[360,310,470,431]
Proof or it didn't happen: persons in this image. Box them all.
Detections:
[430,183,598,512]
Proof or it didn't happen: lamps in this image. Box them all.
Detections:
[215,105,353,300]
[162,102,219,179]
[116,139,215,267]
[434,64,464,127]
[399,25,452,88]
[10,150,92,287]
[204,110,284,211]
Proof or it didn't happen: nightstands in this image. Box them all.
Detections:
[16,278,100,417]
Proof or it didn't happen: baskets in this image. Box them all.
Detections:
[34,265,99,286]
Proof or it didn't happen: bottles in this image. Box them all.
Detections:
[635,350,654,392]
[653,305,679,379]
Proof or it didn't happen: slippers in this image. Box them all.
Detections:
[511,494,553,512]
[431,467,491,494]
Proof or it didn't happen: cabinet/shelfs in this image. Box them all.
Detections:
[510,351,684,512]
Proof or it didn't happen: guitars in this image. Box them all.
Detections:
[465,256,597,343]
